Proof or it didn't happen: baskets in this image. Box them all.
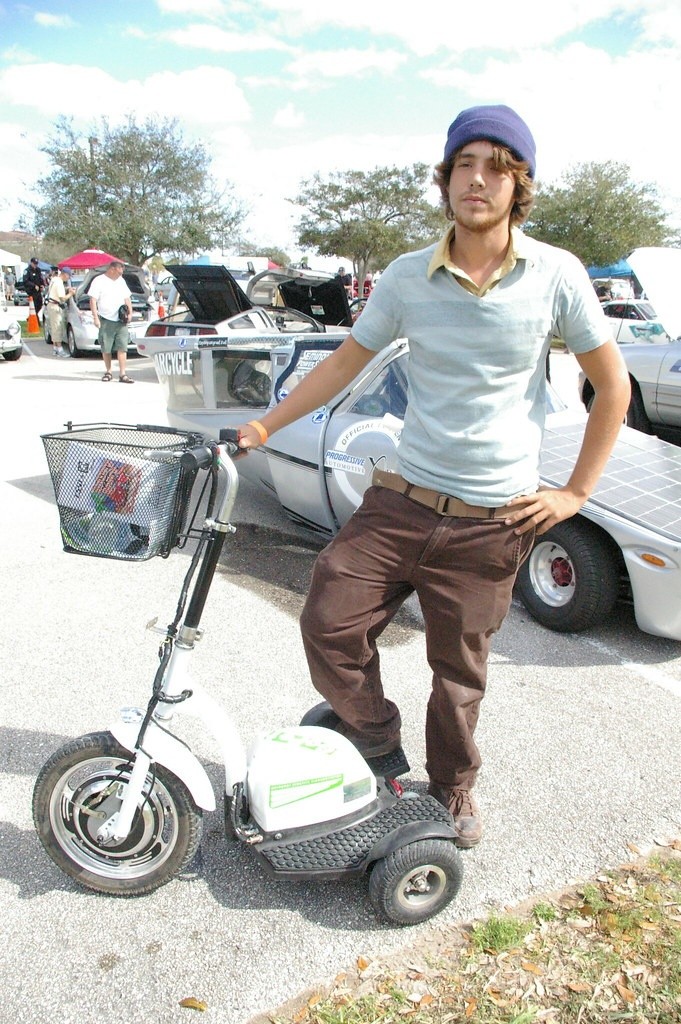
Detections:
[41,424,198,563]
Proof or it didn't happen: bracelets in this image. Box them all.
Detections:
[247,421,268,444]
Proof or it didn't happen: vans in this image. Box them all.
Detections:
[592,278,636,302]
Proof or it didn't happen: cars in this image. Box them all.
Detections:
[42,264,161,358]
[153,275,183,306]
[577,247,681,437]
[602,299,668,345]
[145,258,354,336]
[0,306,23,362]
[14,277,29,306]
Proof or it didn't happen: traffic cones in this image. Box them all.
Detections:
[27,297,40,335]
[158,293,166,320]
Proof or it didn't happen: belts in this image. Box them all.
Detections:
[372,468,528,519]
[49,299,59,306]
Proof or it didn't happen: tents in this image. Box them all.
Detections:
[0,249,52,281]
[584,256,633,278]
[57,245,127,269]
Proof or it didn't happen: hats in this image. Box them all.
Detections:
[62,267,73,277]
[339,267,345,272]
[31,257,39,264]
[7,268,12,272]
[443,104,537,182]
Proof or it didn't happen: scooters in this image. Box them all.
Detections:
[41,420,464,931]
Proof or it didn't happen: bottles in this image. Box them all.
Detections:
[90,511,118,553]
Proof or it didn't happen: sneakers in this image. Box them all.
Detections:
[56,351,70,357]
[429,783,480,846]
[336,720,400,759]
[53,350,56,355]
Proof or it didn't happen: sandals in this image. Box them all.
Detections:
[102,373,112,381]
[119,375,133,383]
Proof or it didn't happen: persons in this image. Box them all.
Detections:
[3,268,15,301]
[45,265,58,286]
[23,257,45,327]
[87,262,135,383]
[45,267,76,358]
[596,280,614,302]
[231,106,632,845]
[335,267,352,294]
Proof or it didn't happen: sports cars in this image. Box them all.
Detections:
[133,331,681,645]
